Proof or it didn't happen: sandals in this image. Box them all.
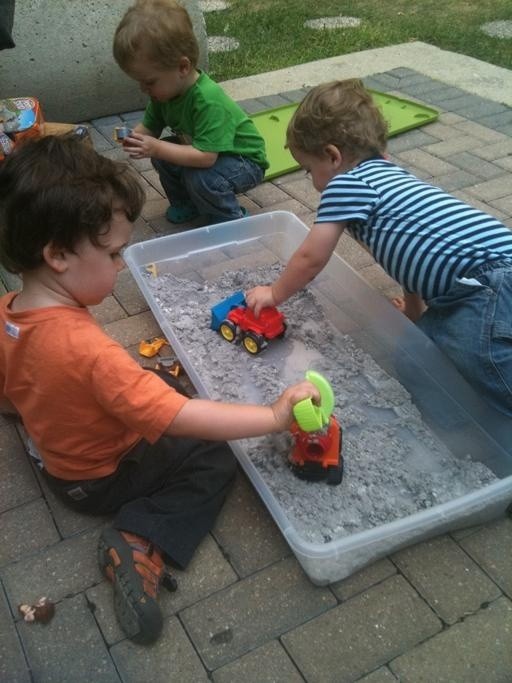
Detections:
[165,203,248,224]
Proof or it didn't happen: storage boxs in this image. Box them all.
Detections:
[123,209,512,589]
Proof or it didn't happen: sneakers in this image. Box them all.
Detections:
[97,526,166,646]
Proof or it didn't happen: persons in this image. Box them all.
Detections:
[239,75,512,436]
[1,125,325,651]
[111,0,272,224]
